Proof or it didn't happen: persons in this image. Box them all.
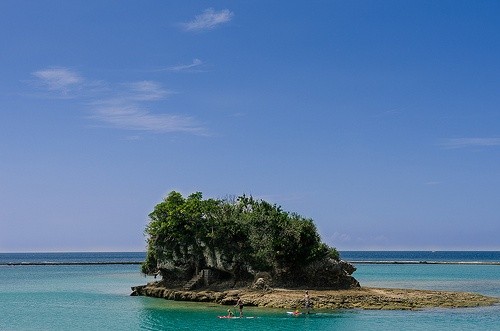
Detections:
[303,290,311,314]
[233,293,244,318]
[218,308,233,318]
